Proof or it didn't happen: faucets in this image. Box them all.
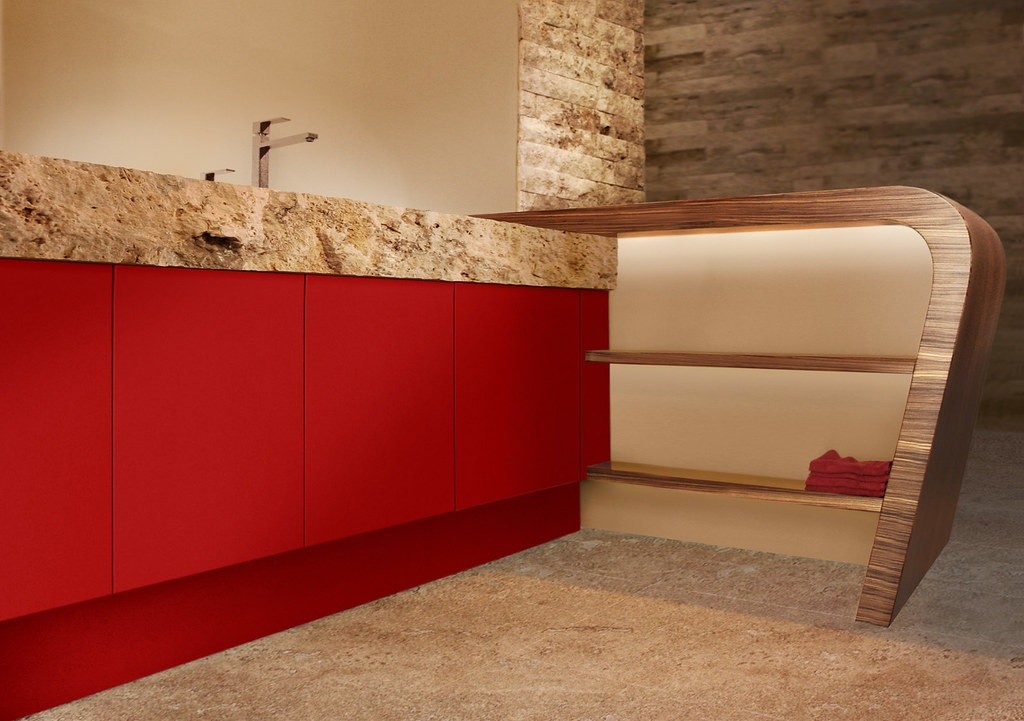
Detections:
[251,116,318,188]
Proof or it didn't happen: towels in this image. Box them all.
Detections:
[804,449,892,498]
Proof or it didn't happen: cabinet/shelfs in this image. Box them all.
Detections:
[469,185,1006,626]
[0,256,612,621]
